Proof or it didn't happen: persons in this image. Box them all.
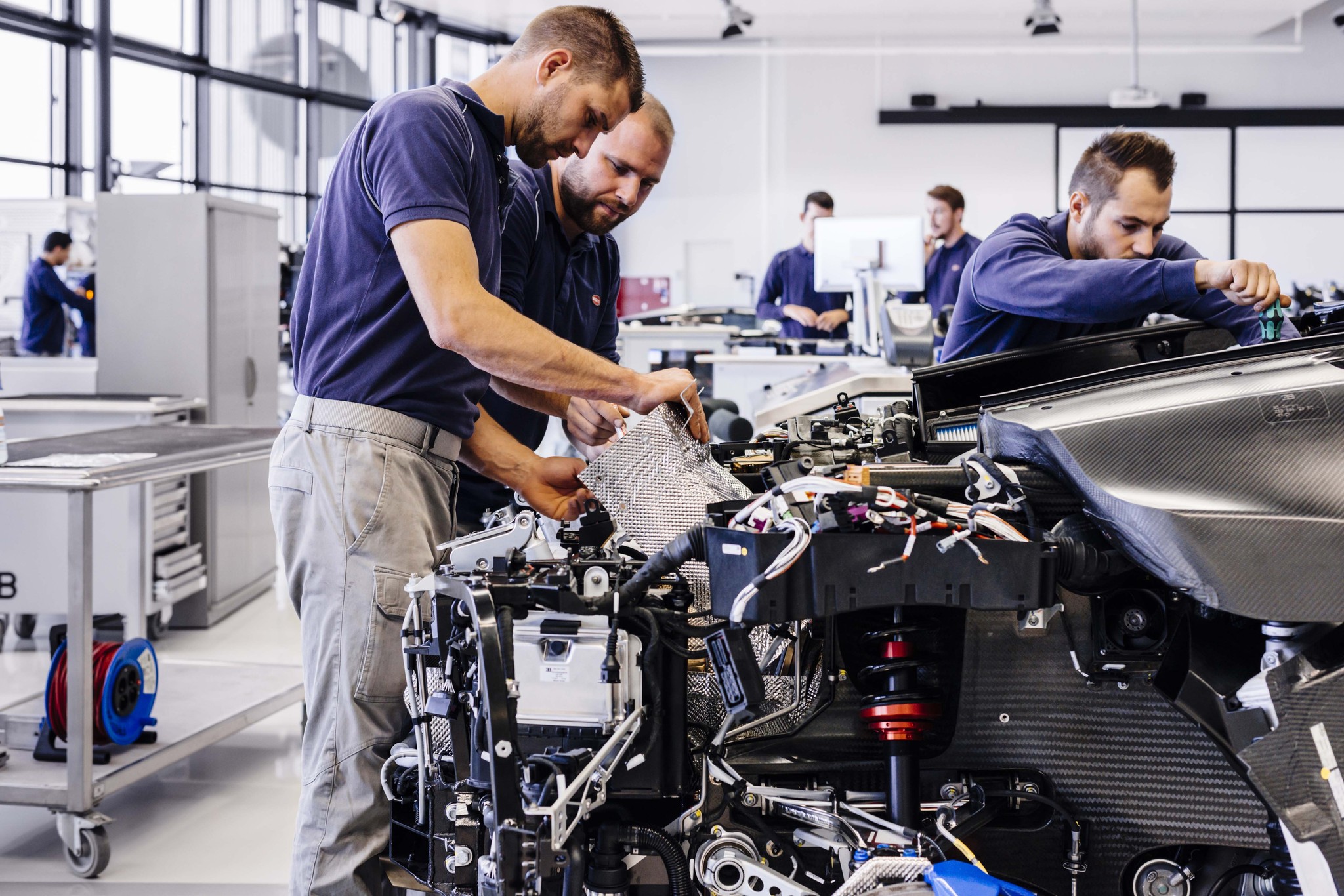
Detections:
[755,191,853,358]
[19,232,96,357]
[449,89,677,538]
[896,185,983,366]
[940,124,1302,368]
[268,4,711,896]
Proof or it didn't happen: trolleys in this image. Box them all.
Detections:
[0,421,309,880]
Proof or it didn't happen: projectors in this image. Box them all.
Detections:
[1107,88,1161,108]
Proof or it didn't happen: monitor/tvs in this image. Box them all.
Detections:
[814,213,927,293]
